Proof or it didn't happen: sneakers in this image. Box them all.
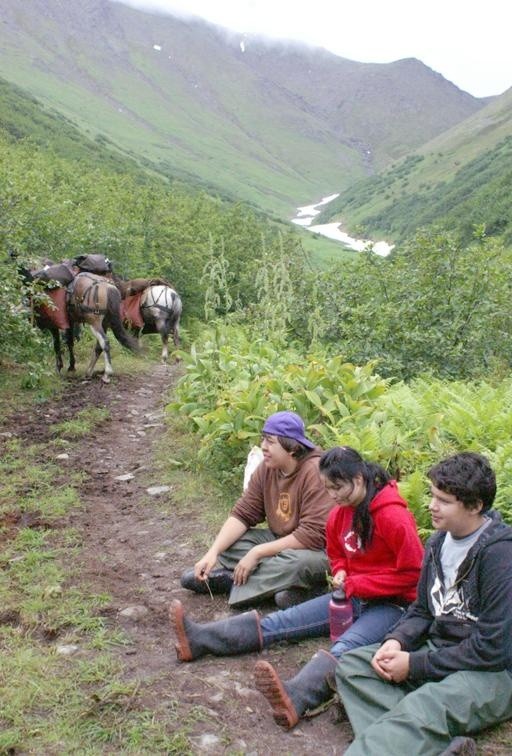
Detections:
[439,736,477,756]
[180,567,232,593]
[273,588,311,609]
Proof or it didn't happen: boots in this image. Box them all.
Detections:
[252,649,339,731]
[168,598,264,661]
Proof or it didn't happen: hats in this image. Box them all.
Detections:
[262,410,316,449]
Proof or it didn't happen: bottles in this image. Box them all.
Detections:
[329,589,353,641]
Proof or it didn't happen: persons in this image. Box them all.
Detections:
[332,451,512,756]
[177,409,336,614]
[169,445,425,732]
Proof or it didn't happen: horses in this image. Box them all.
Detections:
[119,285,184,365]
[17,251,143,384]
